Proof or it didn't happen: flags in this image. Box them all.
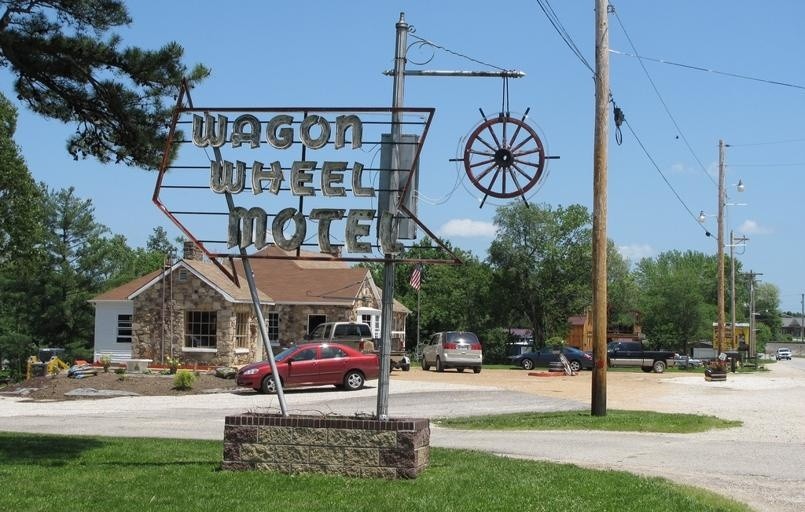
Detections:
[409,261,420,292]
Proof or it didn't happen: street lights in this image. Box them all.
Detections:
[718,139,744,373]
[751,309,769,356]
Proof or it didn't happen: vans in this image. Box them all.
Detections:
[304,322,372,350]
[421,331,482,373]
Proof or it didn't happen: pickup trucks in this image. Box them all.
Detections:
[585,342,674,373]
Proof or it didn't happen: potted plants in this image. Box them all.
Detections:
[165,354,181,374]
[545,336,569,355]
[99,354,111,372]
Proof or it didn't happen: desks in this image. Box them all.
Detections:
[120,359,153,373]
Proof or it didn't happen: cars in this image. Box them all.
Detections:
[512,347,593,371]
[677,356,702,367]
[775,348,791,361]
[236,343,379,390]
